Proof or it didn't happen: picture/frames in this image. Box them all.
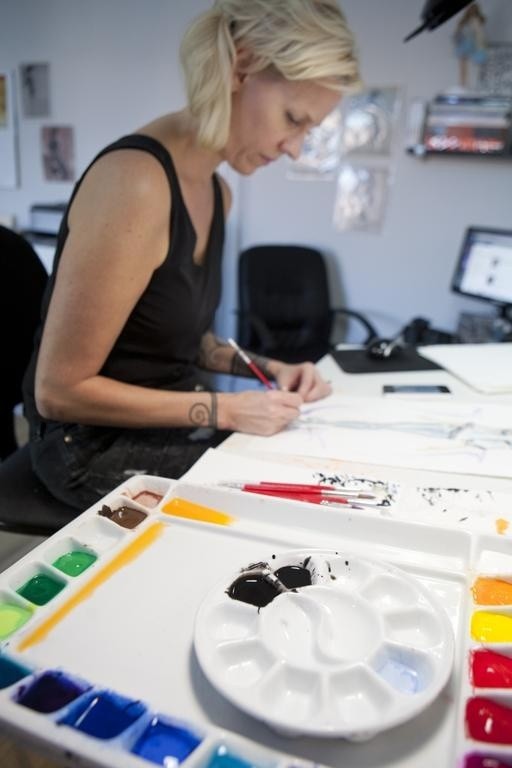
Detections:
[0,62,76,181]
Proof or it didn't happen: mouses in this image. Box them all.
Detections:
[366,338,402,358]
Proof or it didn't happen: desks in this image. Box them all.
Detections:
[0,342,512,768]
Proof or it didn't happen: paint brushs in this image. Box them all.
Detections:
[219,481,390,510]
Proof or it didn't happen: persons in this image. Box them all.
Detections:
[16,0,365,510]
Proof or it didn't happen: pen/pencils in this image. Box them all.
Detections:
[227,338,275,391]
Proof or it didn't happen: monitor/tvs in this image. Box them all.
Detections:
[450,225,512,321]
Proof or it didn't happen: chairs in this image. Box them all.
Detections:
[1,226,102,539]
[224,245,378,392]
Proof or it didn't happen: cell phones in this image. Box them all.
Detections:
[383,385,449,394]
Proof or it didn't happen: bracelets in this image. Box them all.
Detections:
[210,390,218,429]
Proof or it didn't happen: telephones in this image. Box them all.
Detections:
[403,318,452,346]
